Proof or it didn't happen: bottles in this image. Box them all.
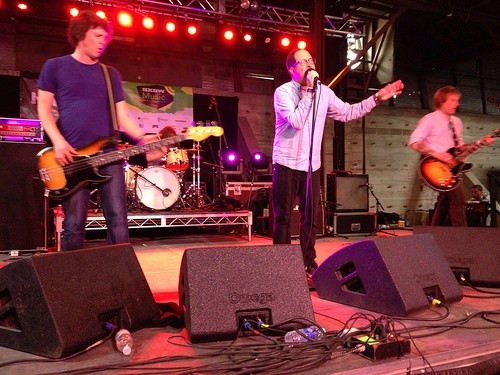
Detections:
[114,329,133,355]
[283,325,326,347]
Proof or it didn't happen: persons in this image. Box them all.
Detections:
[37,10,162,251]
[408,86,494,226]
[273,48,404,279]
[471,185,486,200]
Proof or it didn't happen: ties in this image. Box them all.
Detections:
[449,117,458,145]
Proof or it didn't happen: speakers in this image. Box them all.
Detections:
[0,242,160,359]
[327,173,369,212]
[414,225,500,287]
[312,234,464,317]
[0,141,48,252]
[179,243,315,343]
[268,187,324,237]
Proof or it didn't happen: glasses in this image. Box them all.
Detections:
[292,58,316,66]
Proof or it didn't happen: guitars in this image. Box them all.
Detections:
[417,128,500,194]
[32,120,224,201]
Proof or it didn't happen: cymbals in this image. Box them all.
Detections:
[186,149,205,152]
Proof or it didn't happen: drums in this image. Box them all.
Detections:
[166,147,189,172]
[124,164,144,191]
[135,165,181,210]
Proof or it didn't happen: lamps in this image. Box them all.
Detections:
[239,0,260,18]
[248,151,272,174]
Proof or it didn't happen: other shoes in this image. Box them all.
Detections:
[307,263,318,275]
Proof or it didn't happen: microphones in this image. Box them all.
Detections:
[306,68,320,85]
[208,97,214,110]
[359,183,368,188]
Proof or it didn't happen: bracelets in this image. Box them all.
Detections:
[373,94,381,106]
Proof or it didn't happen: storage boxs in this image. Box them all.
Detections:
[222,181,272,210]
[327,211,377,237]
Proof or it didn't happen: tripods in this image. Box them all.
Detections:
[176,134,213,211]
